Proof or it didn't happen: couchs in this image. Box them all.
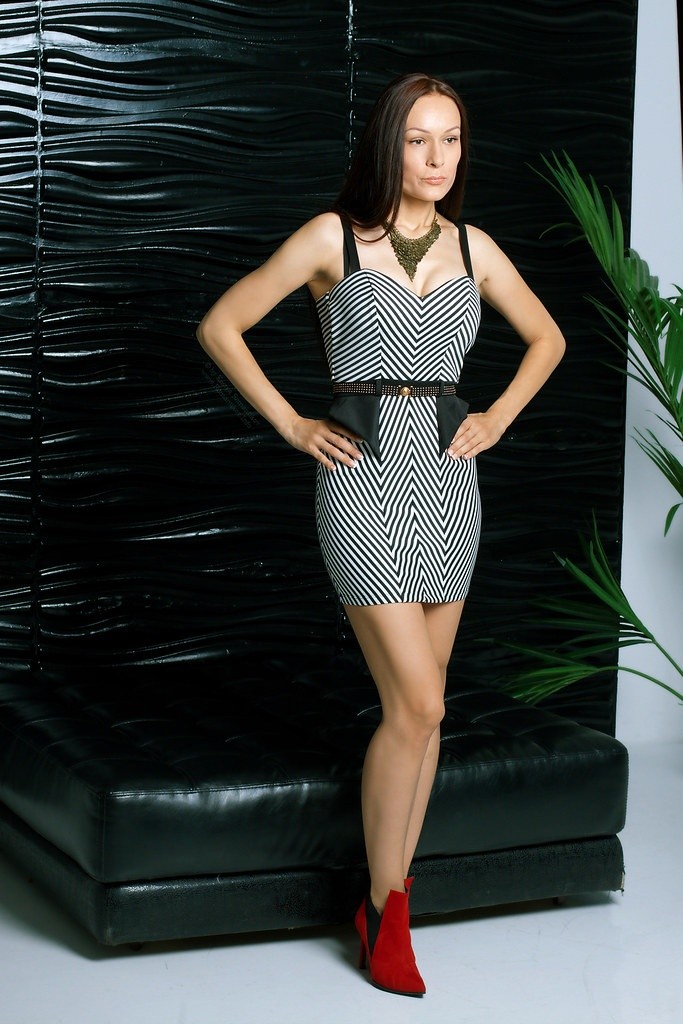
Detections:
[0,671,633,954]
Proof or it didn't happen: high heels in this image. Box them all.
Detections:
[354,875,428,997]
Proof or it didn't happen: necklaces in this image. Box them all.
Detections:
[379,209,442,283]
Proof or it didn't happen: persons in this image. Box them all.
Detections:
[197,73,568,995]
